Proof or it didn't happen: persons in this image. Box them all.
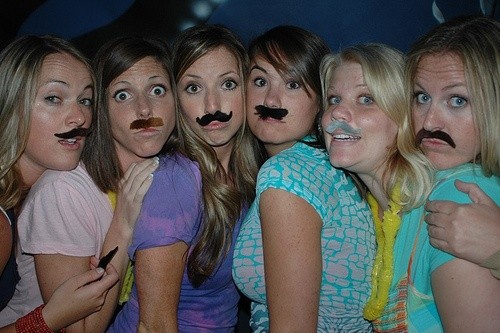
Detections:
[0,33,176,333]
[321,19,499,333]
[231,25,377,333]
[109,25,266,333]
[0,33,119,333]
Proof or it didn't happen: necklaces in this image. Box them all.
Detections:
[363,175,406,321]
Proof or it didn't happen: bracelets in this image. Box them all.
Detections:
[15,304,53,333]
